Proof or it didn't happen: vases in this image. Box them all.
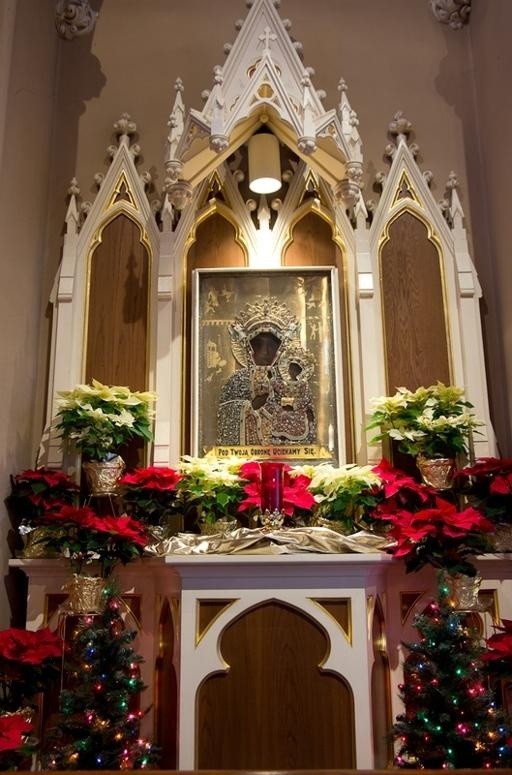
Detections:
[260,461,285,532]
[377,525,396,544]
[198,518,243,537]
[24,524,74,559]
[308,515,355,536]
[0,706,33,752]
[436,568,482,611]
[67,575,112,615]
[82,455,127,498]
[145,523,172,543]
[415,457,456,491]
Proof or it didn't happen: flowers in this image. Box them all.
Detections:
[362,380,486,460]
[481,616,511,669]
[13,466,80,522]
[0,626,74,712]
[31,506,149,574]
[52,378,157,462]
[386,496,501,574]
[452,455,512,494]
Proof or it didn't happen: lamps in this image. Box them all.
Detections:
[246,124,283,196]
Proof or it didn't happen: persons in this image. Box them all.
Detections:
[214,295,320,446]
